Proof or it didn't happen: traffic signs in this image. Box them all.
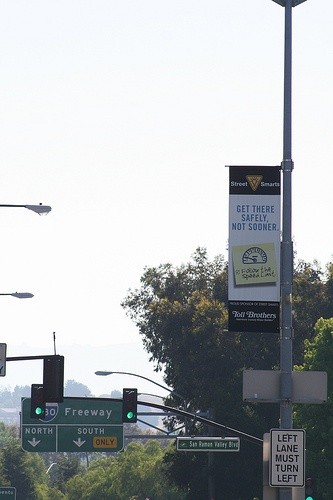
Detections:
[20,396,124,453]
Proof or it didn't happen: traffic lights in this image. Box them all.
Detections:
[31,383,46,417]
[304,477,314,500]
[123,388,138,424]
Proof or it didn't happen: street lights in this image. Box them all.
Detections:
[94,371,217,500]
